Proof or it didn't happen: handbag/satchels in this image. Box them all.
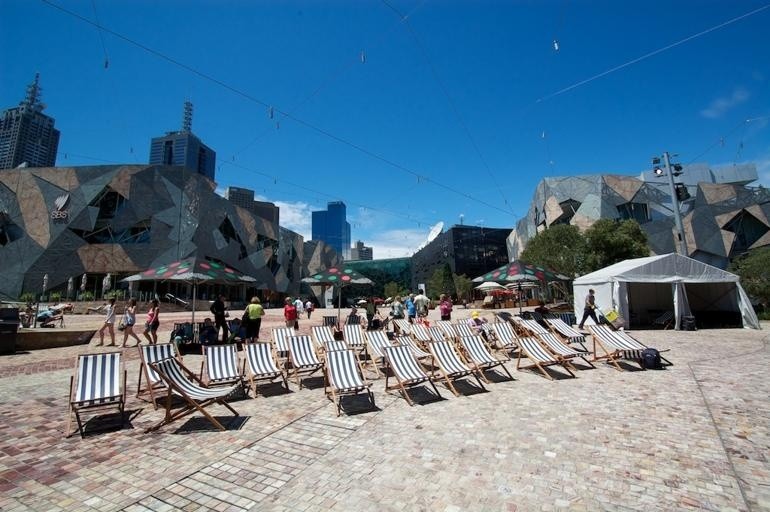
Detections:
[118,320,128,331]
[294,321,298,329]
[242,312,249,325]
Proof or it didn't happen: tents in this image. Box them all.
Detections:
[574,251,762,330]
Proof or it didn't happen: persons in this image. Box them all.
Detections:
[117,298,142,348]
[364,289,454,328]
[579,289,599,329]
[36,309,63,328]
[535,302,552,317]
[143,300,160,346]
[245,297,265,342]
[210,293,229,342]
[202,318,216,344]
[95,296,117,346]
[283,296,313,329]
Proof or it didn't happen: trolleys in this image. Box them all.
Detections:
[594,304,625,329]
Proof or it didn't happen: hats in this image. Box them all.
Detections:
[472,312,478,319]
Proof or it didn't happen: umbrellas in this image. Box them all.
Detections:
[296,266,376,323]
[357,300,367,303]
[475,281,507,296]
[120,252,256,323]
[471,259,570,317]
